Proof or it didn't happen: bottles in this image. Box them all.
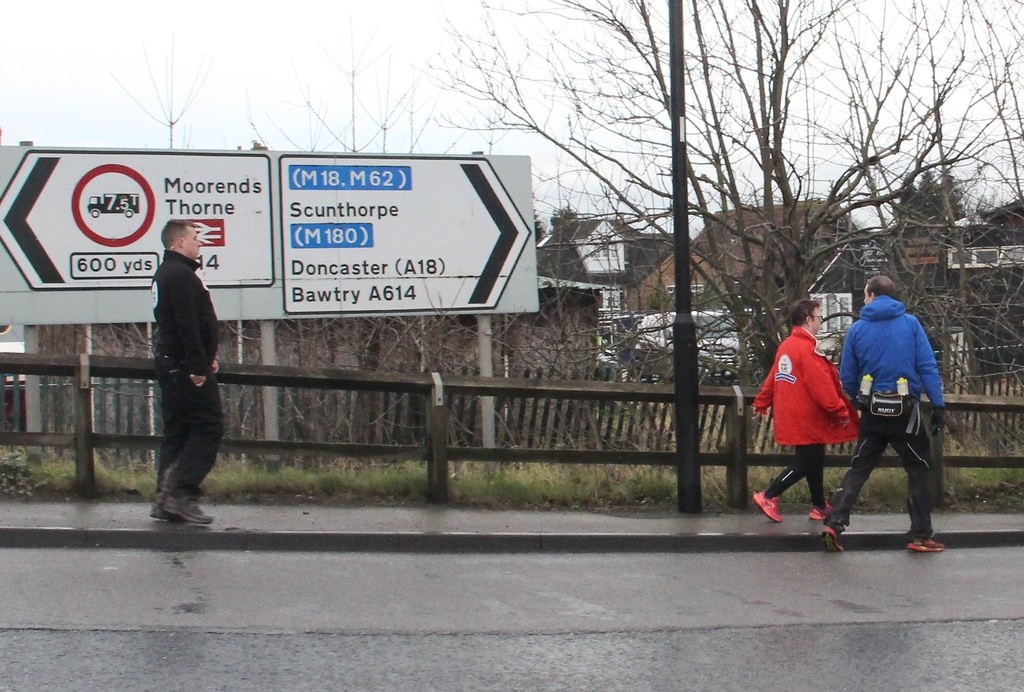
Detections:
[895,377,909,396]
[859,373,874,396]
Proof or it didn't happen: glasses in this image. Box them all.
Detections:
[805,316,824,323]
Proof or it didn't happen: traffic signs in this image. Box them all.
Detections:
[1,147,543,327]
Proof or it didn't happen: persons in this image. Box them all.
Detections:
[820,276,945,551]
[750,299,860,523]
[152,218,225,525]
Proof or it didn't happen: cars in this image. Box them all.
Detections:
[632,310,758,382]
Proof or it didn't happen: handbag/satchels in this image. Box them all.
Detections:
[870,393,904,417]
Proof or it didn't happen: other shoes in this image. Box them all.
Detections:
[163,495,213,524]
[151,499,185,521]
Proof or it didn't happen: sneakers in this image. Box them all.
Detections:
[821,525,844,552]
[810,503,833,520]
[907,535,944,552]
[752,491,783,522]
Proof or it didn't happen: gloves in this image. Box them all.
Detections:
[929,406,945,436]
